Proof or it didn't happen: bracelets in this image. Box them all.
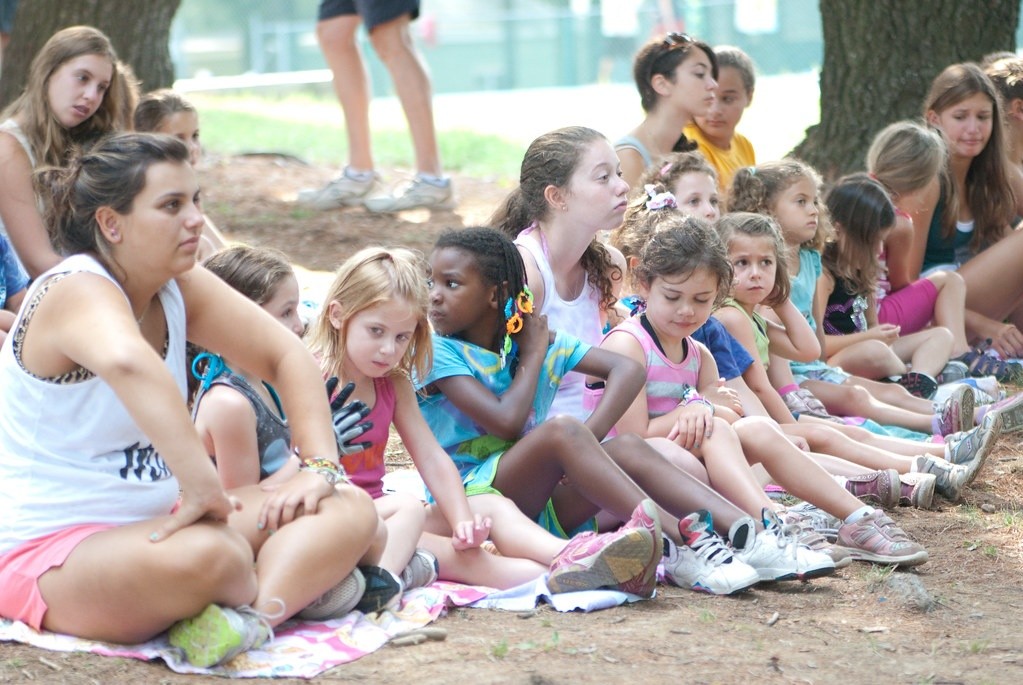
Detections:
[686,398,714,414]
[298,457,350,486]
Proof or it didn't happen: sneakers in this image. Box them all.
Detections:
[776,511,852,570]
[277,567,364,621]
[722,508,839,583]
[837,507,931,567]
[658,510,761,600]
[161,600,258,671]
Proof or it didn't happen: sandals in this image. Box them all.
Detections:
[954,347,1010,383]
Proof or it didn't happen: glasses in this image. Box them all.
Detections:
[657,31,693,49]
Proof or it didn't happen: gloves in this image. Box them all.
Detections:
[323,378,356,413]
[331,401,375,462]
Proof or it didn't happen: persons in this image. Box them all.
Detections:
[864,123,1023,384]
[607,157,1023,508]
[0,232,31,349]
[410,227,836,596]
[187,245,439,613]
[0,26,132,277]
[582,215,929,568]
[303,244,663,598]
[487,127,710,530]
[985,58,1023,171]
[299,0,457,212]
[683,44,755,193]
[0,131,378,668]
[134,89,226,256]
[921,63,1023,362]
[615,32,719,196]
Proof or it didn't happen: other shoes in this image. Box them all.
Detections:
[892,472,940,508]
[362,176,456,216]
[933,384,974,435]
[908,361,969,387]
[285,170,383,217]
[844,467,904,506]
[974,393,1023,434]
[881,372,939,402]
[614,501,666,595]
[541,520,653,595]
[943,409,1004,470]
[916,447,988,502]
[402,548,439,594]
[358,563,407,614]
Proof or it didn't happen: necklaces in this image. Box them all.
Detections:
[138,302,150,323]
[565,262,580,299]
